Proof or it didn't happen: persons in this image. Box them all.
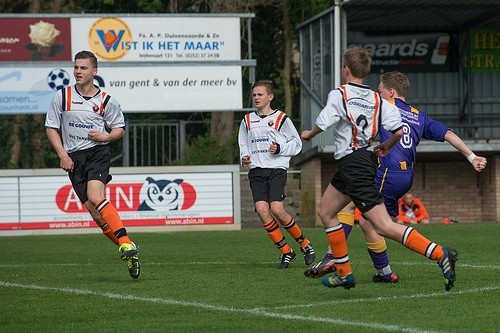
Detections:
[45,50,140,279]
[237,80,315,268]
[301,46,459,292]
[304,71,487,283]
[396,189,430,225]
[354,207,362,225]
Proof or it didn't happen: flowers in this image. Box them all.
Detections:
[25,21,64,61]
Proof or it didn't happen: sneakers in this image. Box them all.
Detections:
[119,241,140,260]
[305,259,336,279]
[321,273,356,289]
[301,242,316,266]
[278,249,296,269]
[127,258,140,279]
[372,272,398,283]
[438,246,458,291]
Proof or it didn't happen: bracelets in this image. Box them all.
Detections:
[467,152,476,164]
